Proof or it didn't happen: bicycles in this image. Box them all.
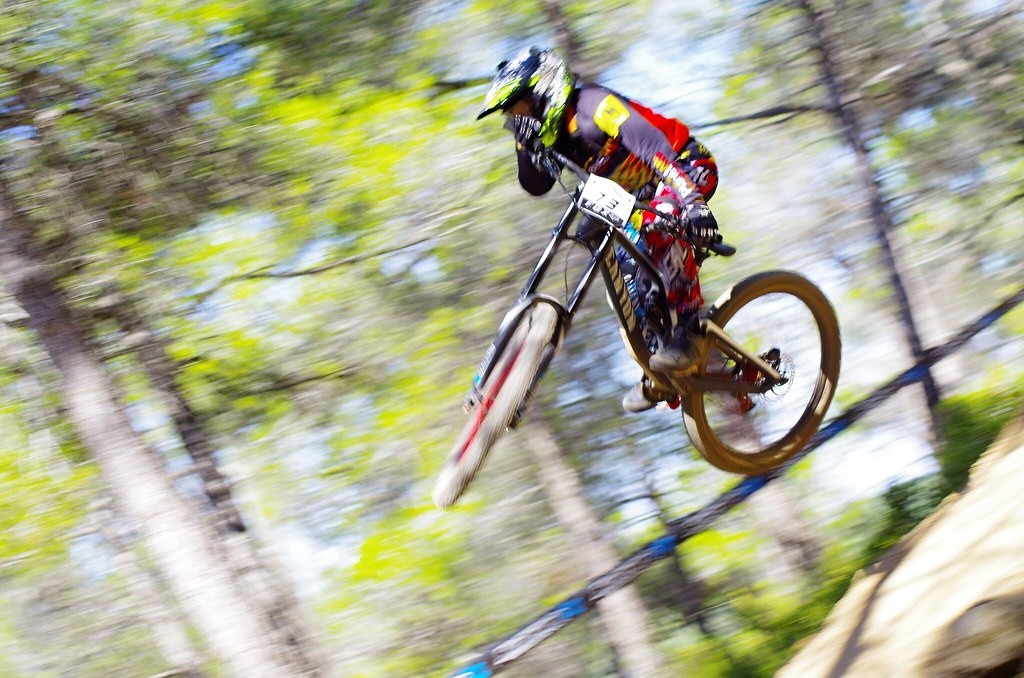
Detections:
[425,115,841,509]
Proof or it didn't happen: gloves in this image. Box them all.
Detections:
[679,206,720,252]
[511,113,542,156]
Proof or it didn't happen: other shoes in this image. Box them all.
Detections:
[622,375,666,412]
[648,325,710,371]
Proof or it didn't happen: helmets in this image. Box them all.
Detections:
[474,44,579,152]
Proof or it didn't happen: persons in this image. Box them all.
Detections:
[475,42,721,411]
